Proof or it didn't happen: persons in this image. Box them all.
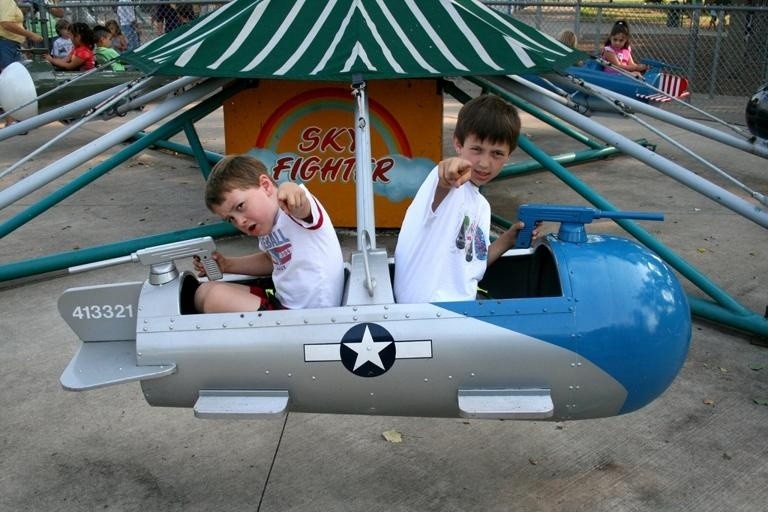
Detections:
[0,0,208,135]
[707,0,760,65]
[193,154,346,312]
[601,20,650,80]
[393,93,545,301]
[558,29,583,67]
